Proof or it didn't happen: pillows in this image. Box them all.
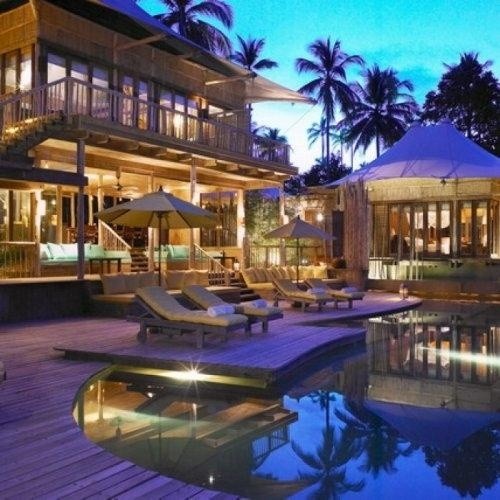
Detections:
[303,265,328,279]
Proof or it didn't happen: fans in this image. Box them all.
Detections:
[94,171,139,191]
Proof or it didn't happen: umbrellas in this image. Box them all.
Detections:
[265,217,339,282]
[91,190,219,288]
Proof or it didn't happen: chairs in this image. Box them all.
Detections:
[93,265,366,350]
